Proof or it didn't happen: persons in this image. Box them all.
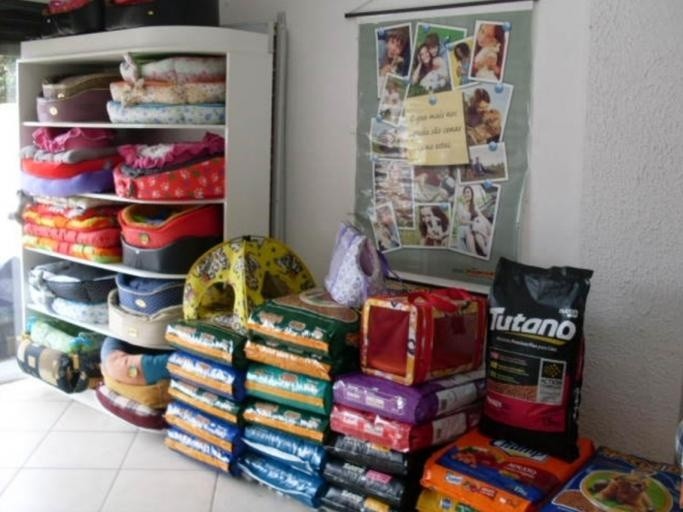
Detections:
[372,23,505,257]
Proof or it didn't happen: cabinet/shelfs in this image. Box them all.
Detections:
[12,25,284,437]
